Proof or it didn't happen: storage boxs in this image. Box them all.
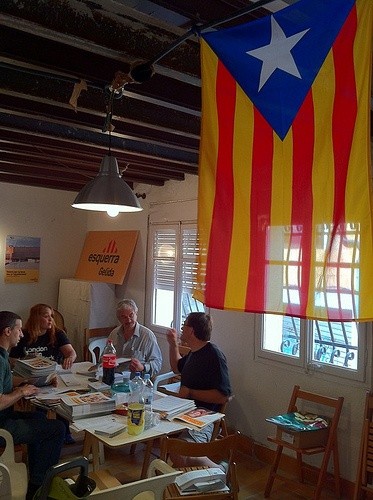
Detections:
[276,423,331,448]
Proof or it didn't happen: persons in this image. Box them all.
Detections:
[165,312,233,473]
[8,303,76,445]
[103,299,162,378]
[0,311,67,500]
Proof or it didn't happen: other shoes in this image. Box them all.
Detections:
[219,459,230,475]
[64,434,76,445]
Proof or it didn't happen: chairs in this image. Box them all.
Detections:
[262,384,343,500]
[0,339,243,500]
[353,391,373,500]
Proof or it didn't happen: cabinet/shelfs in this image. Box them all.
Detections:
[58,279,117,363]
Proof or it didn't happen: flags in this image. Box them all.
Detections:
[193,0,373,323]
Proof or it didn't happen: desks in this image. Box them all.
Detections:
[17,359,193,470]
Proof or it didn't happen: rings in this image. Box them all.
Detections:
[68,361,70,363]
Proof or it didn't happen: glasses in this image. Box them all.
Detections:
[182,324,191,327]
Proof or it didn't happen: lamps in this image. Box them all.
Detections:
[72,65,143,218]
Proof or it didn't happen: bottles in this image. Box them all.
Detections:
[128,373,146,436]
[102,340,116,386]
[142,373,153,430]
